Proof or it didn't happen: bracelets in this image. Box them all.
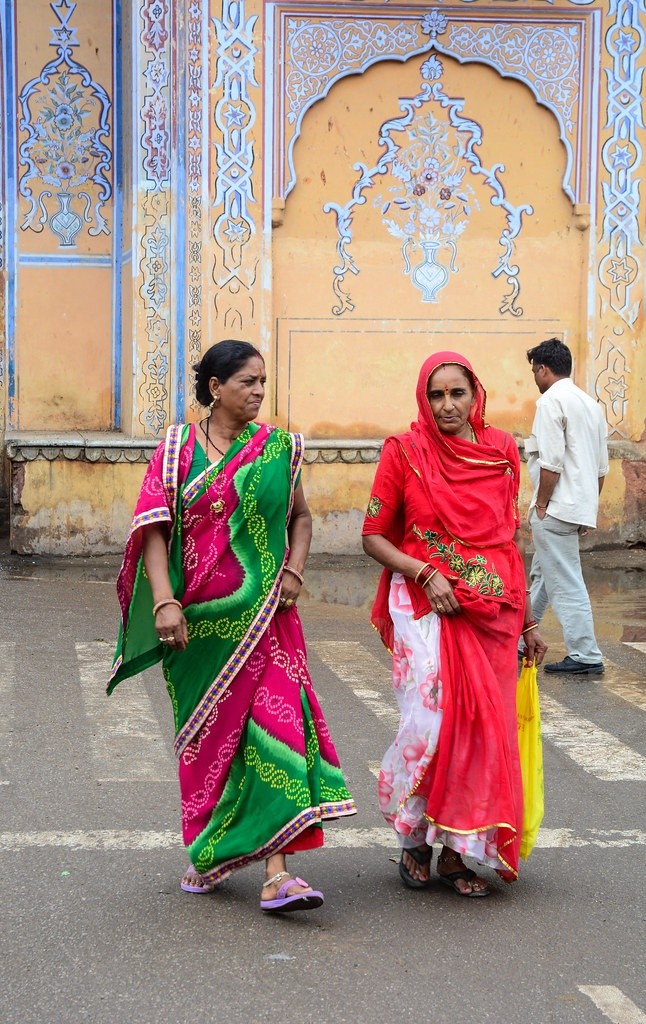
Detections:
[522,620,539,635]
[283,566,305,587]
[415,562,439,589]
[152,598,182,616]
[526,590,531,595]
[534,502,548,510]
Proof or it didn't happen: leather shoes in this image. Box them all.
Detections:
[544,656,607,675]
[517,650,525,658]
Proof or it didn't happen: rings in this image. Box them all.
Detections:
[159,637,166,642]
[279,597,286,603]
[286,599,293,606]
[436,604,443,610]
[167,636,175,641]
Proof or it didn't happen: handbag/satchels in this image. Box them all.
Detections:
[515,656,542,861]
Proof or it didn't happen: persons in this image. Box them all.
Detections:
[518,337,609,674]
[103,340,358,911]
[361,351,549,898]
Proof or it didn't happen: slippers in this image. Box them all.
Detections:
[440,865,492,896]
[397,840,434,887]
[259,876,325,912]
[181,864,217,892]
